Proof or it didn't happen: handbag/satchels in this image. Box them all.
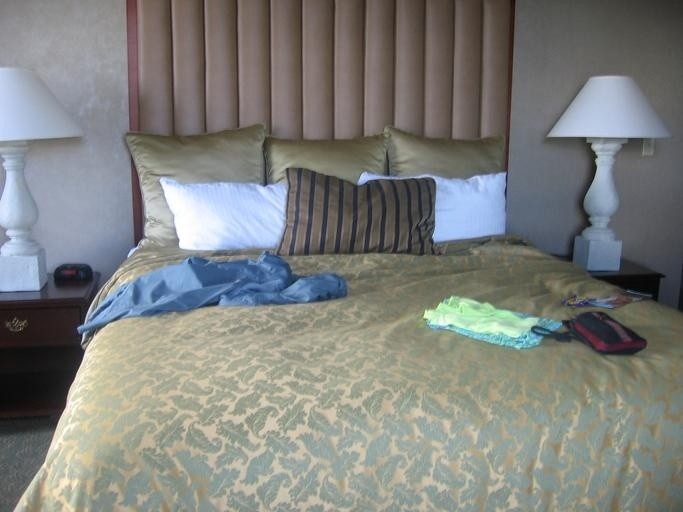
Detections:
[567,312,647,356]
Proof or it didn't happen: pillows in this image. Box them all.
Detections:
[280,166,440,257]
[357,172,511,236]
[123,125,269,244]
[384,128,504,253]
[159,176,291,251]
[261,130,385,187]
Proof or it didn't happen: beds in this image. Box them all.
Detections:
[11,147,682,511]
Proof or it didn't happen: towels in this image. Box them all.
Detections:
[427,291,542,339]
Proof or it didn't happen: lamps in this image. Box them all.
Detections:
[539,75,672,270]
[0,67,90,293]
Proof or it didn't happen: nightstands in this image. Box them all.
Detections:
[0,269,104,424]
[539,256,666,303]
